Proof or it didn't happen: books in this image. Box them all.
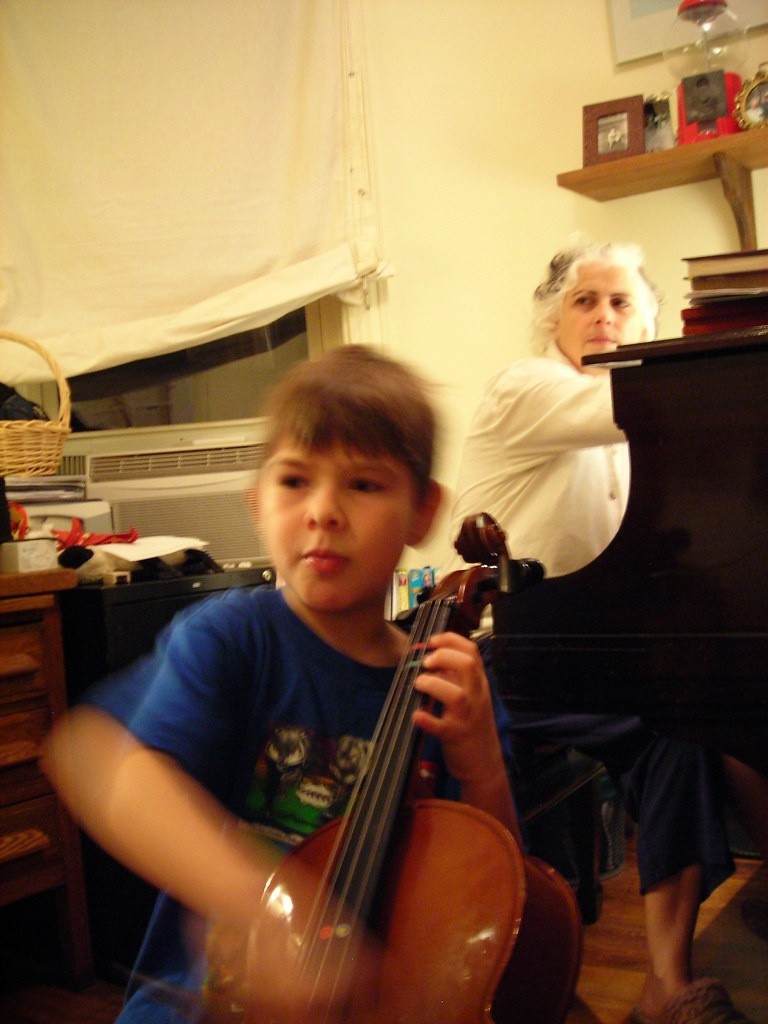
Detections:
[680,247,768,336]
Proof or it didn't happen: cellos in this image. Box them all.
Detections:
[174,511,544,1024]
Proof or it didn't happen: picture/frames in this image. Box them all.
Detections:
[582,94,645,168]
[732,69,768,131]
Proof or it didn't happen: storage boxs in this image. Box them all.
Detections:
[0,538,59,573]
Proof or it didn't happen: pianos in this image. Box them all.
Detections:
[478,312,768,948]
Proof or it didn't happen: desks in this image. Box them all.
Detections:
[0,567,96,996]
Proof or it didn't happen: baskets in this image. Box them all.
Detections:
[0,331,72,477]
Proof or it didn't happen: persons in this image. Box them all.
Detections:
[447,242,751,1024]
[36,346,529,1024]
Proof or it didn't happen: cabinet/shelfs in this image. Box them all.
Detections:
[57,568,276,987]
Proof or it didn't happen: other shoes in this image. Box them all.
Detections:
[633,972,749,1024]
